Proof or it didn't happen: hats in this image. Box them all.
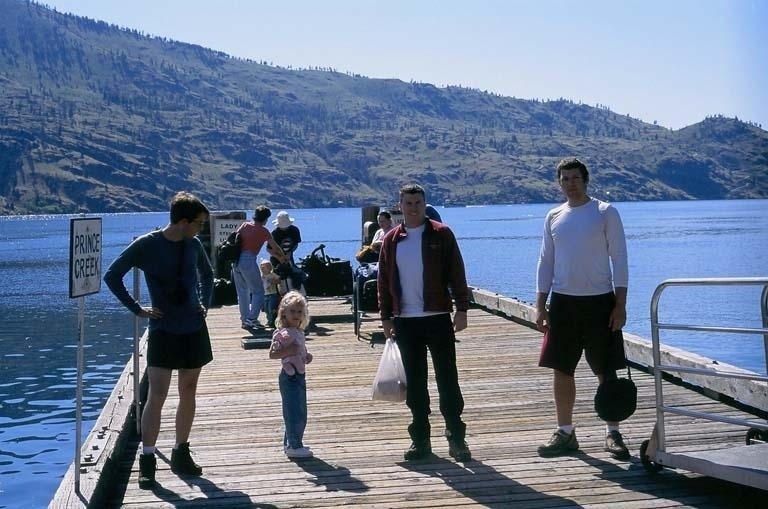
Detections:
[272,210,295,228]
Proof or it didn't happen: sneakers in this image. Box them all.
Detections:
[138,453,156,487]
[537,429,579,455]
[246,318,265,329]
[284,442,313,457]
[406,439,431,460]
[171,442,202,475]
[449,438,471,462]
[602,430,629,459]
[242,323,249,329]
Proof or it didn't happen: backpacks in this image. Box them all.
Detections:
[218,232,242,261]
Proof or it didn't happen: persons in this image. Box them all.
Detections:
[259,261,281,333]
[102,191,215,488]
[376,183,469,463]
[535,156,631,460]
[266,211,307,298]
[229,205,286,331]
[268,290,313,460]
[370,209,394,254]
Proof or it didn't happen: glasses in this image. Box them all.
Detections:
[193,219,205,227]
